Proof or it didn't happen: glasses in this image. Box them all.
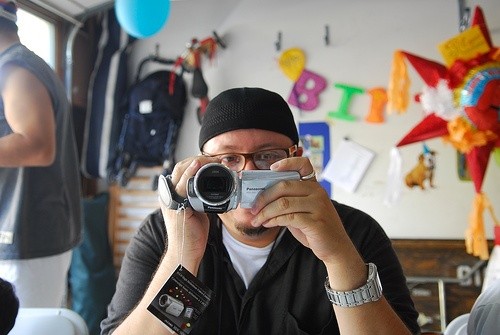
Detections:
[202,144,297,172]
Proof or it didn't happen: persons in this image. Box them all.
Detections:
[0,0,82,309]
[100,87,422,335]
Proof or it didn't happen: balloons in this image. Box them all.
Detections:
[114,0,170,39]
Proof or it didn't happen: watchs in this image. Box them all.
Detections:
[324,263,384,306]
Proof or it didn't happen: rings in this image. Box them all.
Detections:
[301,170,315,180]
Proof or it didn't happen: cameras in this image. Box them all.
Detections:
[186,162,301,214]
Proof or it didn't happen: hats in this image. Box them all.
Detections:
[0,0,18,22]
[198,86,299,150]
